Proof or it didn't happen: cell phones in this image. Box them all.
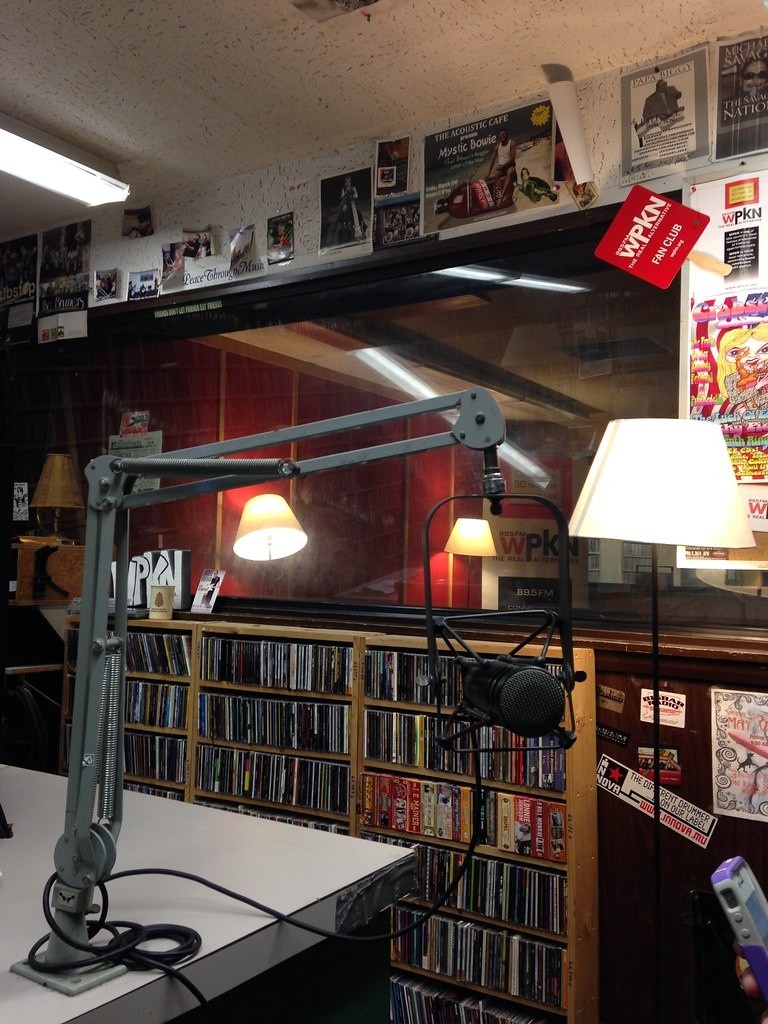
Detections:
[711,857,768,1000]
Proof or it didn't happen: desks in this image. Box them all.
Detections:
[0,761,417,1024]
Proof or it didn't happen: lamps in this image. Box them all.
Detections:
[443,518,498,608]
[0,110,130,207]
[232,493,308,595]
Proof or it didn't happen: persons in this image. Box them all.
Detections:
[721,60,768,129]
[183,233,211,258]
[124,214,152,238]
[95,274,117,295]
[486,130,518,205]
[128,279,158,298]
[42,228,89,296]
[203,569,221,608]
[385,207,421,240]
[336,177,368,241]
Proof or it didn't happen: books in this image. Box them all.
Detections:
[195,744,349,836]
[360,648,565,721]
[120,683,189,729]
[198,693,350,755]
[121,733,185,802]
[361,708,568,793]
[362,833,568,935]
[62,628,194,677]
[200,637,353,696]
[67,595,130,614]
[386,904,568,1023]
[360,772,564,865]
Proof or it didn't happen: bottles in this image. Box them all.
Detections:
[435,176,515,218]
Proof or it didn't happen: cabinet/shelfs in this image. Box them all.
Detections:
[62,615,599,1024]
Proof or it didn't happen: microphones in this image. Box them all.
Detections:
[452,651,569,738]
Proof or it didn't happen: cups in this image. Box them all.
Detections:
[149,585,176,619]
